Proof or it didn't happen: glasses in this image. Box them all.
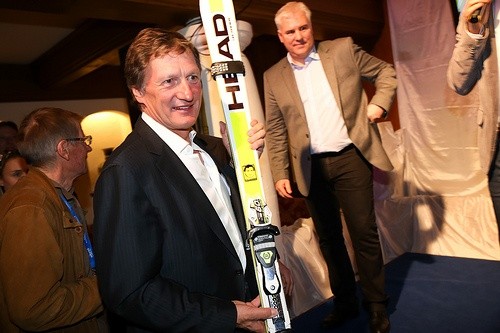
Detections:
[55,136,92,152]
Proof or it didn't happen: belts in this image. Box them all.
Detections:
[310,143,354,159]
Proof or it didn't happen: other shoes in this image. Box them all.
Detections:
[319,308,359,330]
[367,311,391,333]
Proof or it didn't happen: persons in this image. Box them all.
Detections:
[445,0,500,245]
[263,1,397,333]
[0,151,31,194]
[0,107,104,333]
[92,28,292,333]
[0,120,19,164]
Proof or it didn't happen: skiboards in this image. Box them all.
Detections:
[199,0,291,333]
